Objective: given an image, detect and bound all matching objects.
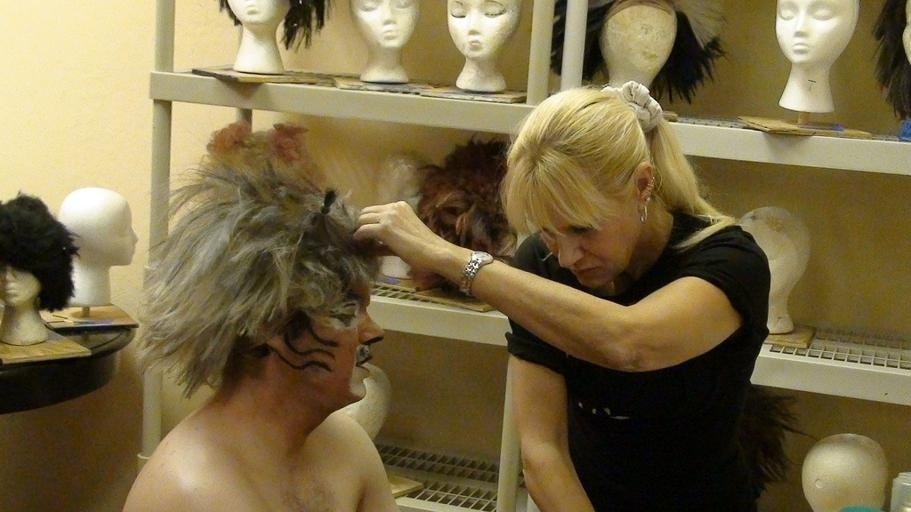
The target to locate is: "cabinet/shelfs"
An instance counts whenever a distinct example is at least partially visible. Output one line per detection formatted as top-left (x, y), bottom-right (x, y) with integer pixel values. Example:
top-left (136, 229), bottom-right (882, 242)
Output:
top-left (134, 1), bottom-right (555, 512)
top-left (536, 0), bottom-right (909, 512)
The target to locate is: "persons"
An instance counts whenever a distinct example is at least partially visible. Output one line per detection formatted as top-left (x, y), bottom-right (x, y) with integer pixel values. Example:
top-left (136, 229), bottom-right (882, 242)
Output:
top-left (123, 148), bottom-right (399, 512)
top-left (347, 2), bottom-right (419, 83)
top-left (551, 2), bottom-right (717, 107)
top-left (354, 82), bottom-right (770, 511)
top-left (219, 0), bottom-right (340, 76)
top-left (876, 0), bottom-right (910, 120)
top-left (775, 1), bottom-right (860, 113)
top-left (0, 195), bottom-right (75, 345)
top-left (445, 0), bottom-right (521, 94)
top-left (408, 141), bottom-right (517, 310)
top-left (741, 204), bottom-right (810, 336)
top-left (58, 185), bottom-right (135, 307)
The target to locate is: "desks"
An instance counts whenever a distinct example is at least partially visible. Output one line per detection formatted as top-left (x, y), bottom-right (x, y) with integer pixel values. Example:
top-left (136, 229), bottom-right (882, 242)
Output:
top-left (0, 304), bottom-right (136, 414)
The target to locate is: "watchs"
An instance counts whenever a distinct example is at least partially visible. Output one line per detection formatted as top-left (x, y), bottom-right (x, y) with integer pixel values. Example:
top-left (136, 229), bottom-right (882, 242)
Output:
top-left (460, 249), bottom-right (493, 296)
